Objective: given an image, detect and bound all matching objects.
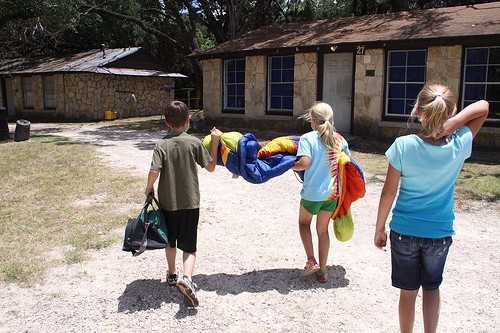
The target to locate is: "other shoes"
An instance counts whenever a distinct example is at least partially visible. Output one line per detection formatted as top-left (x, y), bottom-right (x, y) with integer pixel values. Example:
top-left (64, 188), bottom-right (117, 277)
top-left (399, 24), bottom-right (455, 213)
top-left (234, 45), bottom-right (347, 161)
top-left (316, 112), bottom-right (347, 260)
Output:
top-left (166, 266), bottom-right (180, 286)
top-left (176, 275), bottom-right (199, 307)
top-left (301, 257), bottom-right (320, 277)
top-left (316, 269), bottom-right (328, 283)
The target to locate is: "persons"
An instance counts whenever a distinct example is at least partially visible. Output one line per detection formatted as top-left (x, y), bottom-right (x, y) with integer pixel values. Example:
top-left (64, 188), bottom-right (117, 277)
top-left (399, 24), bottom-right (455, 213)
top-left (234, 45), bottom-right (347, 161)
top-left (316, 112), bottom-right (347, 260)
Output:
top-left (288, 103), bottom-right (351, 283)
top-left (145, 101), bottom-right (223, 308)
top-left (374, 83), bottom-right (489, 333)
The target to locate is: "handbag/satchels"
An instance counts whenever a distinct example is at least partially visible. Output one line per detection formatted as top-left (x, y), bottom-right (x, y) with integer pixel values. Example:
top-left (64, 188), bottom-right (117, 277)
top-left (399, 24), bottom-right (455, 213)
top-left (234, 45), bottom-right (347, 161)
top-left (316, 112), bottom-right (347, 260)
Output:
top-left (122, 192), bottom-right (168, 257)
top-left (333, 205), bottom-right (354, 242)
